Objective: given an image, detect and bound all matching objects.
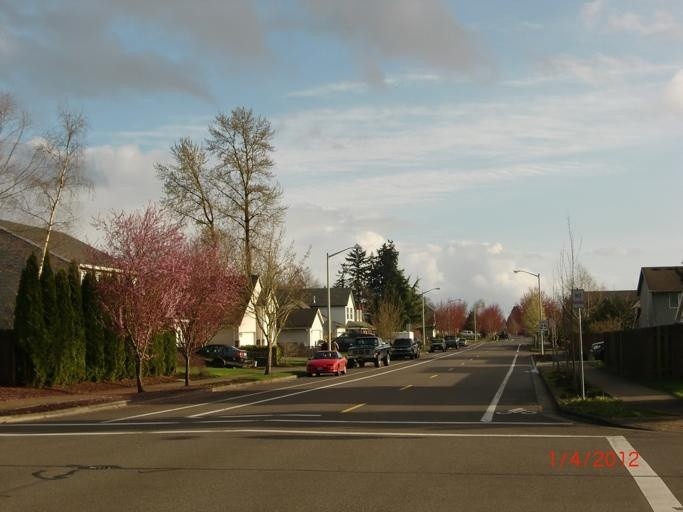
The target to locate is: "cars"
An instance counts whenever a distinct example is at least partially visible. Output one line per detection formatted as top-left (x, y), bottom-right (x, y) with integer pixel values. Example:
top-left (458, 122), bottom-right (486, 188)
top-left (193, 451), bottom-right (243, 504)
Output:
top-left (590, 341), bottom-right (604, 360)
top-left (194, 343), bottom-right (248, 369)
top-left (498, 331), bottom-right (509, 340)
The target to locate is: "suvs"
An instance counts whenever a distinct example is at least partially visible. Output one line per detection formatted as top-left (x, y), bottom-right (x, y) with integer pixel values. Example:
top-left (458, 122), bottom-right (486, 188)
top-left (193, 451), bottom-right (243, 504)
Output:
top-left (304, 330), bottom-right (481, 378)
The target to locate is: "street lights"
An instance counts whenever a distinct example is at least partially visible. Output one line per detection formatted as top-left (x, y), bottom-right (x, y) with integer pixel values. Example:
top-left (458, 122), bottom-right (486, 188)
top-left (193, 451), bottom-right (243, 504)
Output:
top-left (325, 245), bottom-right (361, 356)
top-left (513, 268), bottom-right (540, 356)
top-left (448, 299), bottom-right (461, 337)
top-left (421, 287), bottom-right (441, 345)
